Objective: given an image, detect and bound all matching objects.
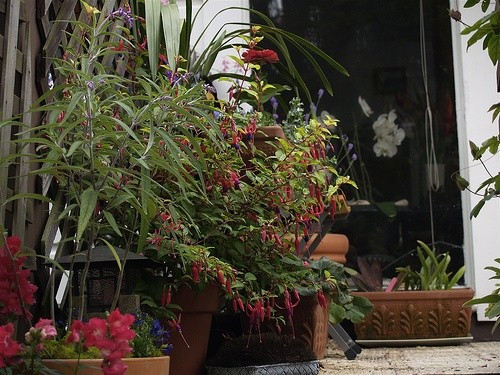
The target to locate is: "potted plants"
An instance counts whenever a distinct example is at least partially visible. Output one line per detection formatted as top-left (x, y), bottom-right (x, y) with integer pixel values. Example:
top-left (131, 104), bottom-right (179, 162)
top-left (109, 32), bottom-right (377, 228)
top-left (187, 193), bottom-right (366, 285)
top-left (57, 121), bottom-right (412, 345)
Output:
top-left (346, 239), bottom-right (474, 347)
